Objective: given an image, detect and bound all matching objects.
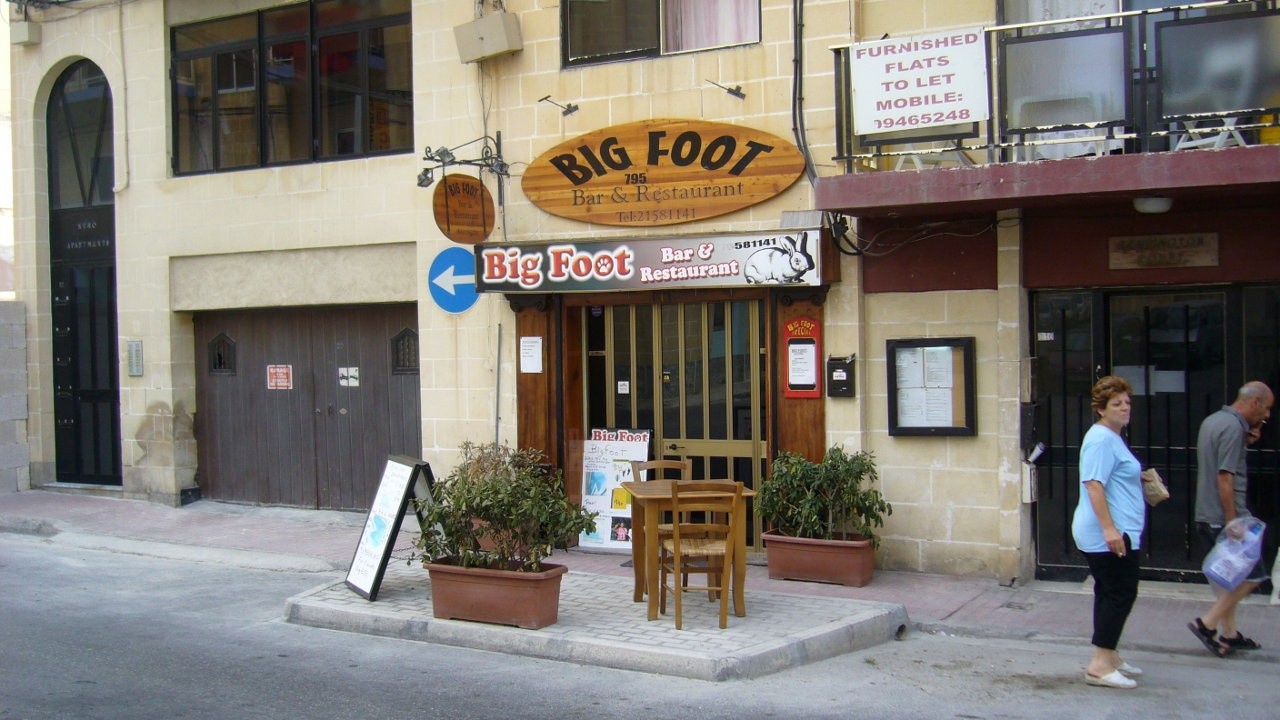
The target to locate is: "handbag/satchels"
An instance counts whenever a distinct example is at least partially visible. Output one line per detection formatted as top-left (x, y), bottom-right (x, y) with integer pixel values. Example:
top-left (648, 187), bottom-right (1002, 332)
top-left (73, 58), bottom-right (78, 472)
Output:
top-left (1202, 516), bottom-right (1267, 592)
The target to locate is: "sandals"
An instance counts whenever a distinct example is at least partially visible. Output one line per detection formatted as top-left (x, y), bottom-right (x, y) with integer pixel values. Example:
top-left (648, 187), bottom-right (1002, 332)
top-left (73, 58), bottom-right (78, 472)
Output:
top-left (1187, 616), bottom-right (1228, 659)
top-left (1218, 630), bottom-right (1262, 651)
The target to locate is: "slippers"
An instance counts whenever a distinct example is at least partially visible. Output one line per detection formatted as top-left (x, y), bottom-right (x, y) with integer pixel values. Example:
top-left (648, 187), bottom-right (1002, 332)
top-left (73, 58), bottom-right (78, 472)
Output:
top-left (1085, 669), bottom-right (1137, 689)
top-left (1115, 661), bottom-right (1143, 678)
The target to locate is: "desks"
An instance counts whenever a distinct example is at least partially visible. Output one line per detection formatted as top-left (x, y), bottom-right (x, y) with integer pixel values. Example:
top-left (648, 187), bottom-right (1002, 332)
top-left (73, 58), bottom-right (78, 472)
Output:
top-left (620, 479), bottom-right (759, 621)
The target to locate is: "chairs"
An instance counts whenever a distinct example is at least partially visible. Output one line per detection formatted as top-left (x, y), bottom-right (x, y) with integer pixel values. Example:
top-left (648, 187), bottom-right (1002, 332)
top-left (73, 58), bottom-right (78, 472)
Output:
top-left (627, 457), bottom-right (744, 629)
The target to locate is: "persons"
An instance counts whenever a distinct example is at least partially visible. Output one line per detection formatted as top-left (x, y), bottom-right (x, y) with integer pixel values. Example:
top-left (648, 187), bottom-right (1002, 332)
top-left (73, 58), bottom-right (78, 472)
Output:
top-left (1072, 375), bottom-right (1163, 689)
top-left (1186, 382), bottom-right (1275, 658)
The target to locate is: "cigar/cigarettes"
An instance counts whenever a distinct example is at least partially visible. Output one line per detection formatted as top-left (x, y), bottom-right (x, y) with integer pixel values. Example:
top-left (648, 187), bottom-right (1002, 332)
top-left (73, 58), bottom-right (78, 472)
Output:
top-left (1262, 420), bottom-right (1266, 423)
top-left (1119, 553), bottom-right (1122, 556)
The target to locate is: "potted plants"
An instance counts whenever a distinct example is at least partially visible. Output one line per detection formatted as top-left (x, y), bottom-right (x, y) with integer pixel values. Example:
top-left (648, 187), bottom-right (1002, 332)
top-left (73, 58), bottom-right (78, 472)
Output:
top-left (751, 442), bottom-right (893, 587)
top-left (407, 439), bottom-right (599, 628)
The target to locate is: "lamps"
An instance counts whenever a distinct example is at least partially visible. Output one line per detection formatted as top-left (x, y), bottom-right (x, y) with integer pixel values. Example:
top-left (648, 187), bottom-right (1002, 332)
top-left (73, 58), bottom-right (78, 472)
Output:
top-left (416, 130), bottom-right (509, 206)
top-left (1133, 198), bottom-right (1173, 212)
